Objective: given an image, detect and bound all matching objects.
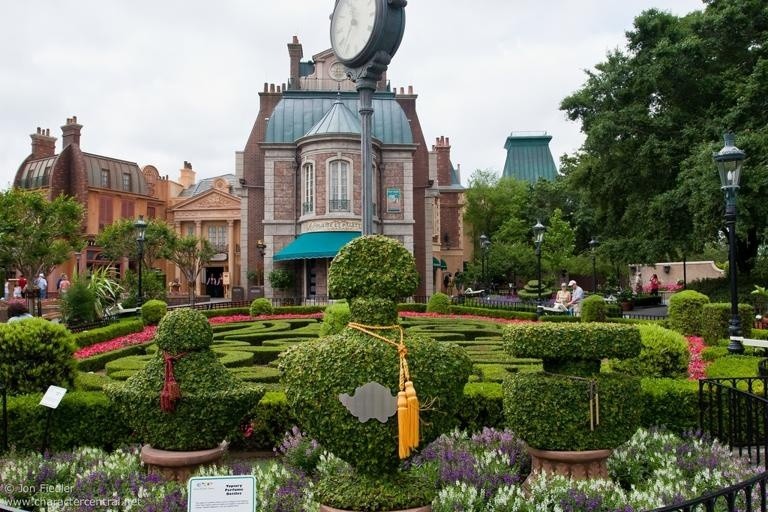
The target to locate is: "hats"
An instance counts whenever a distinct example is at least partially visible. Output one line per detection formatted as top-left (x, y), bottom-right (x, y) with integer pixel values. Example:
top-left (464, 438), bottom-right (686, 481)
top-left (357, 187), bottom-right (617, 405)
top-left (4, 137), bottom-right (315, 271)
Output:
top-left (561, 283), bottom-right (567, 286)
top-left (568, 280), bottom-right (576, 286)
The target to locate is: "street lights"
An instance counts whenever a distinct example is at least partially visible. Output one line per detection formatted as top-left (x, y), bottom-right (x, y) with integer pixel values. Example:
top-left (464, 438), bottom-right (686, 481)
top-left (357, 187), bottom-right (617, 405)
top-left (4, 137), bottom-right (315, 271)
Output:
top-left (713, 131), bottom-right (747, 356)
top-left (478, 233), bottom-right (487, 281)
top-left (255, 239), bottom-right (266, 288)
top-left (484, 240), bottom-right (490, 281)
top-left (531, 218), bottom-right (545, 318)
top-left (133, 215), bottom-right (147, 306)
top-left (74, 251), bottom-right (82, 279)
top-left (679, 246), bottom-right (686, 292)
top-left (585, 235), bottom-right (598, 294)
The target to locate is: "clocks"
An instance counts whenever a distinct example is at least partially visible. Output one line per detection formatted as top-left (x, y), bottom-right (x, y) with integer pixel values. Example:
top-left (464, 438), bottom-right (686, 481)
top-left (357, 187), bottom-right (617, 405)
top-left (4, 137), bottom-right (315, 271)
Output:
top-left (328, 0), bottom-right (406, 234)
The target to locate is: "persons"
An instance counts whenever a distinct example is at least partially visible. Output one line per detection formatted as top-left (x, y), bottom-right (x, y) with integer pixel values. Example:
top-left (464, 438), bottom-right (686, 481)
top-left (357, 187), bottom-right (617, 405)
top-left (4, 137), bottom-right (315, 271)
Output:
top-left (448, 272), bottom-right (454, 296)
top-left (565, 279), bottom-right (584, 317)
top-left (649, 274), bottom-right (659, 296)
top-left (34, 273), bottom-right (47, 298)
top-left (59, 275), bottom-right (71, 293)
top-left (18, 274), bottom-right (27, 298)
top-left (443, 275), bottom-right (449, 294)
top-left (454, 268), bottom-right (464, 295)
top-left (556, 283), bottom-right (572, 315)
top-left (56, 272), bottom-right (66, 295)
top-left (634, 272), bottom-right (644, 293)
